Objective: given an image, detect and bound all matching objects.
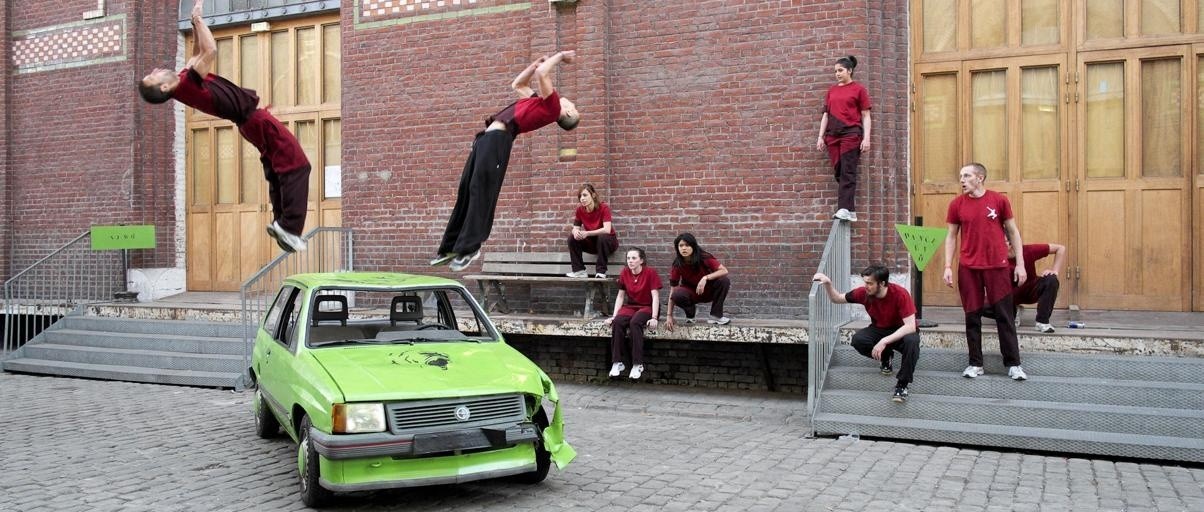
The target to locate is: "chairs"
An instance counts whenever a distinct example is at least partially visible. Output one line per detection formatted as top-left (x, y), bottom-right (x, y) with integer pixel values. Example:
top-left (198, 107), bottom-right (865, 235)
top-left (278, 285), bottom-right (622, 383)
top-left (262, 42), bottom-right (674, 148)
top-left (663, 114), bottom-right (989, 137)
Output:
top-left (380, 295), bottom-right (440, 331)
top-left (308, 295), bottom-right (369, 345)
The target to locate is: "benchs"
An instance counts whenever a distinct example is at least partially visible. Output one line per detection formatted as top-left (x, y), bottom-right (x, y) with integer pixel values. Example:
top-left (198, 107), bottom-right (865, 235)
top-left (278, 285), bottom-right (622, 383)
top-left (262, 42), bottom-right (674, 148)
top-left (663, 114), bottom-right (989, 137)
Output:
top-left (462, 251), bottom-right (627, 320)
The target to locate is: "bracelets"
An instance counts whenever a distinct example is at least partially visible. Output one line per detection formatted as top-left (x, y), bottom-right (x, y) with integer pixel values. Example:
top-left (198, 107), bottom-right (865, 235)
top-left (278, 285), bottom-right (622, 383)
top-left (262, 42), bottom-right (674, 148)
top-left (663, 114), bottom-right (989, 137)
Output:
top-left (944, 265), bottom-right (952, 269)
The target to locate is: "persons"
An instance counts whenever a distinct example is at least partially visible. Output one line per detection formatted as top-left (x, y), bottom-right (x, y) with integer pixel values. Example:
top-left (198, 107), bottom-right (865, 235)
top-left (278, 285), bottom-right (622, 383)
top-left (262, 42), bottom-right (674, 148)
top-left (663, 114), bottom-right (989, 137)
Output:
top-left (568, 183), bottom-right (619, 279)
top-left (601, 249), bottom-right (662, 381)
top-left (811, 264), bottom-right (919, 406)
top-left (140, 1), bottom-right (311, 254)
top-left (975, 228), bottom-right (1065, 333)
top-left (943, 162), bottom-right (1029, 383)
top-left (431, 48), bottom-right (580, 272)
top-left (817, 55), bottom-right (871, 222)
top-left (666, 232), bottom-right (732, 331)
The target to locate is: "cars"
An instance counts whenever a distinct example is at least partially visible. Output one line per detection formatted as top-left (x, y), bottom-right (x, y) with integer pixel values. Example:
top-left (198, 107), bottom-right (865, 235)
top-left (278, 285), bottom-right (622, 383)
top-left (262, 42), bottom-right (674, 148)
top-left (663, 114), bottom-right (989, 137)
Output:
top-left (246, 271), bottom-right (577, 506)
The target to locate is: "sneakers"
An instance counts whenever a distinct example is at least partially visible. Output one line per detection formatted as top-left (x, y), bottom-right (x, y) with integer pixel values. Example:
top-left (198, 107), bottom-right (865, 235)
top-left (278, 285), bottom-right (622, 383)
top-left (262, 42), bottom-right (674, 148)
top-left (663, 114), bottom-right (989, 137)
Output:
top-left (628, 365), bottom-right (644, 379)
top-left (449, 248), bottom-right (481, 272)
top-left (962, 365), bottom-right (985, 378)
top-left (707, 312), bottom-right (731, 325)
top-left (267, 221), bottom-right (305, 253)
top-left (1035, 321), bottom-right (1054, 333)
top-left (1007, 366), bottom-right (1027, 380)
top-left (832, 208), bottom-right (857, 222)
top-left (566, 270), bottom-right (588, 278)
top-left (595, 273), bottom-right (606, 278)
top-left (609, 362), bottom-right (625, 377)
top-left (881, 351), bottom-right (894, 374)
top-left (893, 386), bottom-right (908, 402)
top-left (430, 252), bottom-right (459, 266)
top-left (686, 317), bottom-right (695, 323)
top-left (1014, 305), bottom-right (1024, 327)
